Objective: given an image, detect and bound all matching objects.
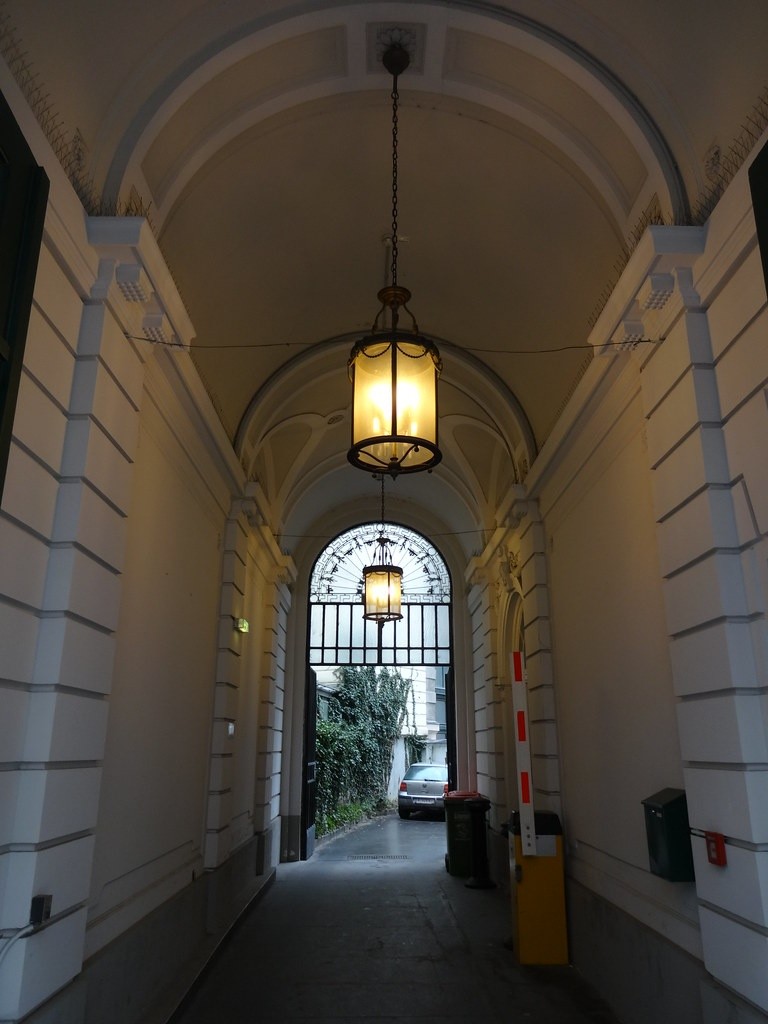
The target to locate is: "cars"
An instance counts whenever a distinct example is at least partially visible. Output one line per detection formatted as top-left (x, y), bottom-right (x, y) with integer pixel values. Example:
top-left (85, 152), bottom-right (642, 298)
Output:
top-left (398, 760), bottom-right (450, 818)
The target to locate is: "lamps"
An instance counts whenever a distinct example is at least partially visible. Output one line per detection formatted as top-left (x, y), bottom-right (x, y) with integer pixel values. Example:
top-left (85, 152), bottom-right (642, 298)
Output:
top-left (234, 618), bottom-right (249, 634)
top-left (363, 473), bottom-right (404, 627)
top-left (346, 28), bottom-right (443, 481)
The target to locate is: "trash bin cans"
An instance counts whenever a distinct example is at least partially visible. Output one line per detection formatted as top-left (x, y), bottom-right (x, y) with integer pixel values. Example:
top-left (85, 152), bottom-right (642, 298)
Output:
top-left (441, 791), bottom-right (492, 878)
